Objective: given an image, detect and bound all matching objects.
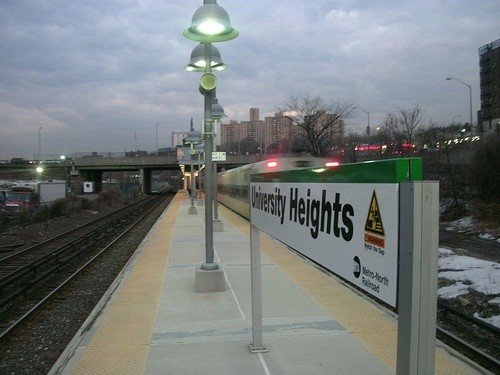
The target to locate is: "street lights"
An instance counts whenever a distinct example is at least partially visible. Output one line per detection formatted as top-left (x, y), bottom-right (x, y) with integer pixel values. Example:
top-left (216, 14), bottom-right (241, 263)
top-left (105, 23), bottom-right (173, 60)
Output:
top-left (445, 77), bottom-right (473, 142)
top-left (450, 115), bottom-right (463, 145)
top-left (352, 106), bottom-right (371, 161)
top-left (183, 0), bottom-right (240, 293)
top-left (38, 126), bottom-right (42, 161)
top-left (155, 122), bottom-right (160, 154)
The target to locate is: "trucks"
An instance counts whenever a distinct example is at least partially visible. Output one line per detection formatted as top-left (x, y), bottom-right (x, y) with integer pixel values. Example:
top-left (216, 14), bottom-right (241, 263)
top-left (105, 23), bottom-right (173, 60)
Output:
top-left (0, 178), bottom-right (66, 224)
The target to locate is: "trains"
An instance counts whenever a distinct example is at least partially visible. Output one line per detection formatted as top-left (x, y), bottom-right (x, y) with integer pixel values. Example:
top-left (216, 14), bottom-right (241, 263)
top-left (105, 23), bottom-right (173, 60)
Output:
top-left (195, 156), bottom-right (341, 221)
